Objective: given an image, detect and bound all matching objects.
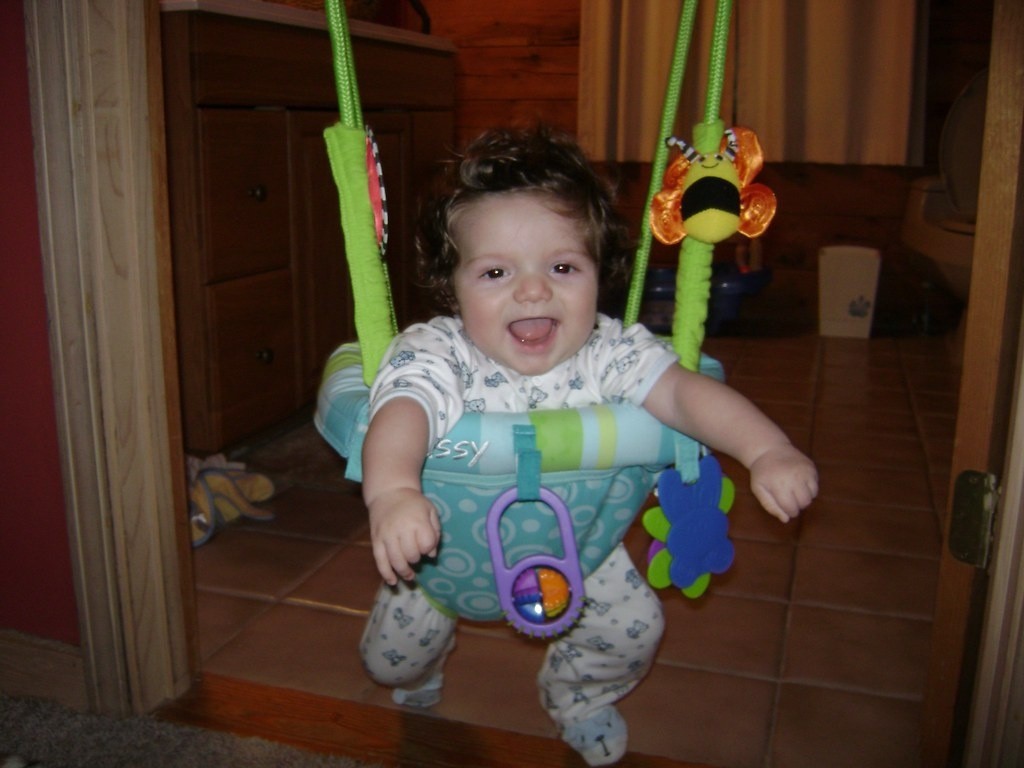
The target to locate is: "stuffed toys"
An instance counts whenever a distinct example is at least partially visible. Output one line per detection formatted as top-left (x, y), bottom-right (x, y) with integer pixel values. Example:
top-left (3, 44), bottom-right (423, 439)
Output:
top-left (651, 128), bottom-right (777, 244)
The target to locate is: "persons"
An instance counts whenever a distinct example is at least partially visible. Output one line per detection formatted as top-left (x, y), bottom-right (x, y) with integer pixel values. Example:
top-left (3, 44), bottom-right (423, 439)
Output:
top-left (360, 126), bottom-right (818, 768)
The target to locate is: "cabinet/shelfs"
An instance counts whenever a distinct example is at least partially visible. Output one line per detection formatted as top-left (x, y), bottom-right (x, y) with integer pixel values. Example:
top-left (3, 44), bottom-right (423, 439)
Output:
top-left (401, 110), bottom-right (459, 325)
top-left (283, 111), bottom-right (412, 411)
top-left (194, 104), bottom-right (307, 455)
